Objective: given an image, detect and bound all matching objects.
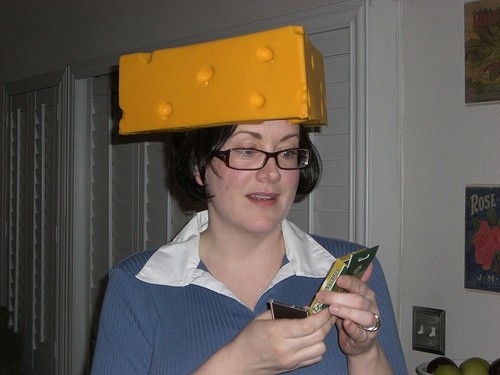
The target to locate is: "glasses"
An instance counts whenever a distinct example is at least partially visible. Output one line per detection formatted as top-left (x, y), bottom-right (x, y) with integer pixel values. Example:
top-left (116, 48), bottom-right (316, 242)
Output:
top-left (215, 146), bottom-right (311, 170)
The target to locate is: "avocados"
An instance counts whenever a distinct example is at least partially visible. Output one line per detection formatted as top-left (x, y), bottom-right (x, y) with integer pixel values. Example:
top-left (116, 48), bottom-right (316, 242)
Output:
top-left (425, 356), bottom-right (500, 375)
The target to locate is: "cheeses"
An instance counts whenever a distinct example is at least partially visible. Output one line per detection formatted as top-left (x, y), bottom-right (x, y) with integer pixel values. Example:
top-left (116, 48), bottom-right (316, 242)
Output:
top-left (117, 25), bottom-right (327, 134)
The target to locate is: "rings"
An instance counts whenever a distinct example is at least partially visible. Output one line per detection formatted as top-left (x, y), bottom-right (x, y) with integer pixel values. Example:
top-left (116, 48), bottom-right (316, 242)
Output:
top-left (363, 314), bottom-right (380, 331)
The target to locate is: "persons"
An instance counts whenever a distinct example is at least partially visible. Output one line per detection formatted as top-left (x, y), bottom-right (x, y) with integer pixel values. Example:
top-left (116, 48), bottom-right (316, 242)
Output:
top-left (90, 118), bottom-right (408, 375)
